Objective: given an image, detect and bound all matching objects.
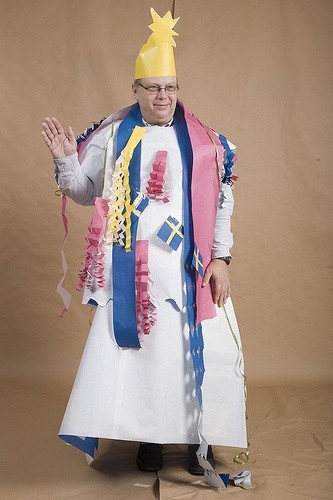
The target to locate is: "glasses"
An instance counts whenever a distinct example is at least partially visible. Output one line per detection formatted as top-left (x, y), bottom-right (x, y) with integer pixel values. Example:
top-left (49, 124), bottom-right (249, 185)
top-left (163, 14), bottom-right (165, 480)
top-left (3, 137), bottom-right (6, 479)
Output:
top-left (136, 82), bottom-right (180, 94)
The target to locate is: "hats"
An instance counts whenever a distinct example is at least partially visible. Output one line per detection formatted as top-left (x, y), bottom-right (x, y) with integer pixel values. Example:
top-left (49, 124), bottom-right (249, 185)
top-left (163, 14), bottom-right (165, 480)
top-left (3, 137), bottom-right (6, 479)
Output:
top-left (134, 8), bottom-right (180, 81)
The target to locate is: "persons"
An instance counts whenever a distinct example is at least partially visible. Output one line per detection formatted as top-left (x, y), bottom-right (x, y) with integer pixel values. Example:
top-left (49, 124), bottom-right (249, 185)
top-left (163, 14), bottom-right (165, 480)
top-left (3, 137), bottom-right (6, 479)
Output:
top-left (41, 6), bottom-right (247, 476)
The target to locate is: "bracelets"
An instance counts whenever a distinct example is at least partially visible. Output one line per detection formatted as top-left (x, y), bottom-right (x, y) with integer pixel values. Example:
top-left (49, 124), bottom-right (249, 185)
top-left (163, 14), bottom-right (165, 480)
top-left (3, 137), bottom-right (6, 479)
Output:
top-left (211, 255), bottom-right (231, 265)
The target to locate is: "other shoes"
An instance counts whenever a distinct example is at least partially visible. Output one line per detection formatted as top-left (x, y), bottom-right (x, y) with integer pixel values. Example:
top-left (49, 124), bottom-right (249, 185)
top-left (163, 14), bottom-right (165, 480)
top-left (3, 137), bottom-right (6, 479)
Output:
top-left (188, 444), bottom-right (216, 475)
top-left (137, 442), bottom-right (165, 472)
